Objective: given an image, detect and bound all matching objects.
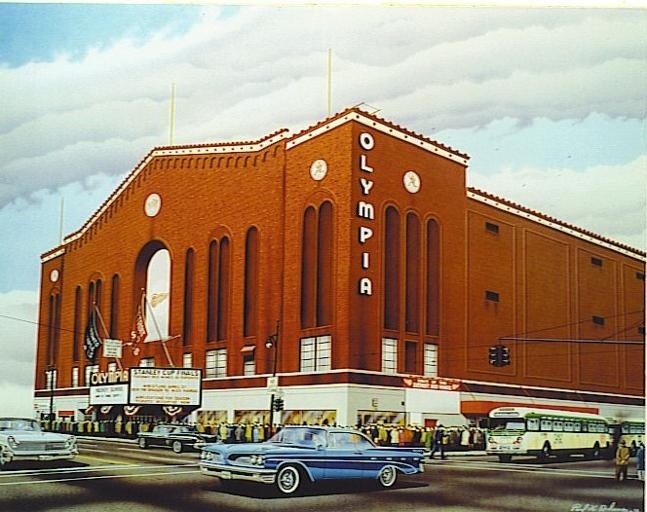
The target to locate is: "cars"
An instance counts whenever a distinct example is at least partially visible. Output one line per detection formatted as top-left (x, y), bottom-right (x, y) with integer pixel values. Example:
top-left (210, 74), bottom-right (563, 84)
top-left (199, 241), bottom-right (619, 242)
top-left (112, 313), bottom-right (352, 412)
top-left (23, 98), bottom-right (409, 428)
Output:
top-left (137, 422), bottom-right (221, 455)
top-left (198, 424), bottom-right (430, 489)
top-left (0, 415), bottom-right (80, 470)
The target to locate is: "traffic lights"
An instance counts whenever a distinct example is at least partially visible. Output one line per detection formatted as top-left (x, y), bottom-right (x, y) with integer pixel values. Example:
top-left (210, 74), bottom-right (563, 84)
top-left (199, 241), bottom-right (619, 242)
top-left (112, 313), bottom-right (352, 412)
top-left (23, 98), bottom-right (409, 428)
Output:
top-left (499, 346), bottom-right (510, 366)
top-left (488, 345), bottom-right (498, 366)
top-left (273, 398), bottom-right (285, 413)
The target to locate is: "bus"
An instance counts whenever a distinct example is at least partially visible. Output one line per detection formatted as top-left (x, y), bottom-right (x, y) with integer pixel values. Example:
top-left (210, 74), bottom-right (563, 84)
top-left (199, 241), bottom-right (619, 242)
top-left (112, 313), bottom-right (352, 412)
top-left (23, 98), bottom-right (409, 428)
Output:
top-left (485, 407), bottom-right (610, 460)
top-left (602, 415), bottom-right (645, 456)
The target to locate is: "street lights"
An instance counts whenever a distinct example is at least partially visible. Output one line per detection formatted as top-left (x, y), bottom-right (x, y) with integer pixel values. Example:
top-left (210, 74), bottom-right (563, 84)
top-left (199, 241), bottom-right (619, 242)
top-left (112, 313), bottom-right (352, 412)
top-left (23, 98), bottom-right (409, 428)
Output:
top-left (265, 319), bottom-right (281, 440)
top-left (45, 365), bottom-right (58, 430)
